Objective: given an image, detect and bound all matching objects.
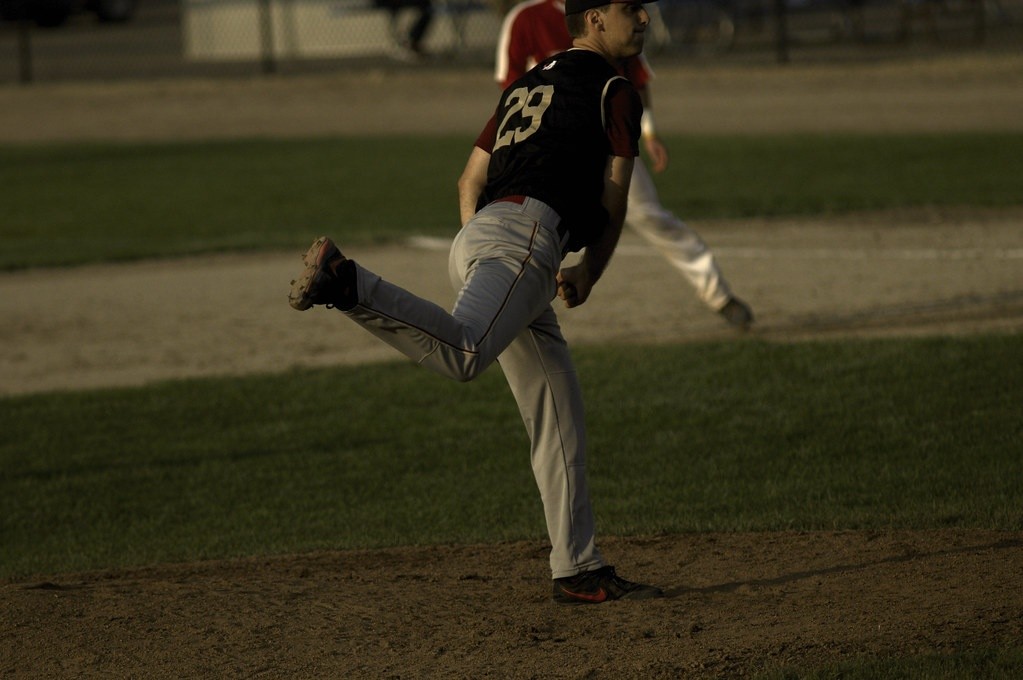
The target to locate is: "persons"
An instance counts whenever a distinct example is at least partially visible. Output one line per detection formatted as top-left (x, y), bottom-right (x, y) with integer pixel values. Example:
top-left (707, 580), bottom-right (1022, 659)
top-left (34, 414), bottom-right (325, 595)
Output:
top-left (495, 0), bottom-right (753, 329)
top-left (288, 0), bottom-right (665, 607)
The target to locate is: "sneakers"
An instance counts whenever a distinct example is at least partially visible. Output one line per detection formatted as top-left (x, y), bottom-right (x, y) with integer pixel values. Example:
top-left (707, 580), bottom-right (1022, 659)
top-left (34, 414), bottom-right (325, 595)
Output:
top-left (552, 564), bottom-right (663, 604)
top-left (287, 236), bottom-right (347, 311)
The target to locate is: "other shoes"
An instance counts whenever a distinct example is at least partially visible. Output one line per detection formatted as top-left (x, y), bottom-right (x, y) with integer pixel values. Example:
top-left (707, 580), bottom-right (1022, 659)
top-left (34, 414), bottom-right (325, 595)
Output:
top-left (721, 298), bottom-right (755, 331)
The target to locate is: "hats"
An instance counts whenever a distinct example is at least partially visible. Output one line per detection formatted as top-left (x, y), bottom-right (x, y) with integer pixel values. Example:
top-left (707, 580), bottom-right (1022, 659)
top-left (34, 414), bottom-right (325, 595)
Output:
top-left (565, 0), bottom-right (659, 16)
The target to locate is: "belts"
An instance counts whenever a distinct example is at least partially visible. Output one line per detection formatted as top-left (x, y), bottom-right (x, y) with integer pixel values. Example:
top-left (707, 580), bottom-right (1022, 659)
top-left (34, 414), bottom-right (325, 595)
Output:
top-left (494, 194), bottom-right (567, 241)
